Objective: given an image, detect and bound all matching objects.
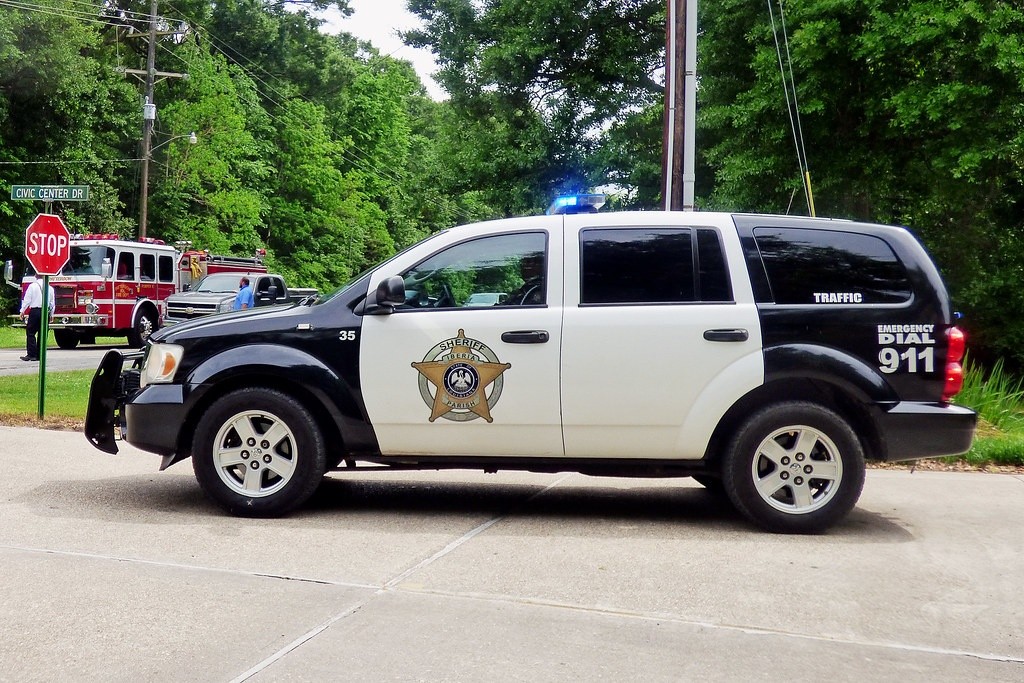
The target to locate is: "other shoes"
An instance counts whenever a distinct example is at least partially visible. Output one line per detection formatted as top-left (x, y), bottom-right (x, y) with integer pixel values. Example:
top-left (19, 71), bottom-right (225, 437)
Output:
top-left (21, 355), bottom-right (36, 361)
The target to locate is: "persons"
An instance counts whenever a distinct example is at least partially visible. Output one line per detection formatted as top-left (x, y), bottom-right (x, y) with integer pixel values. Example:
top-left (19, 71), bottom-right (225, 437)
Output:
top-left (494, 252), bottom-right (544, 306)
top-left (20, 273), bottom-right (56, 361)
top-left (234, 277), bottom-right (254, 310)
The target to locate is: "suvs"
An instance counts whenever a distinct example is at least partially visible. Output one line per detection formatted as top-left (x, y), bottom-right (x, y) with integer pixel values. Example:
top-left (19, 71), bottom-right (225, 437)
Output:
top-left (85, 193), bottom-right (982, 536)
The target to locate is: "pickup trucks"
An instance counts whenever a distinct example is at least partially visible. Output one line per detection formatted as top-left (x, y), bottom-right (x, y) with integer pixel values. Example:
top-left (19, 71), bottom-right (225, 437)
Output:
top-left (158, 272), bottom-right (320, 331)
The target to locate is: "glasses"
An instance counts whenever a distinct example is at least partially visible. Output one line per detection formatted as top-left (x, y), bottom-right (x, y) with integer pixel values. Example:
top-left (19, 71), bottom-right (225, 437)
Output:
top-left (519, 260), bottom-right (537, 268)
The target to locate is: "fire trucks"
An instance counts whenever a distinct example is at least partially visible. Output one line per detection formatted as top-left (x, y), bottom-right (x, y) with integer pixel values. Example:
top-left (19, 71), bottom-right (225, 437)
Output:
top-left (4, 233), bottom-right (268, 350)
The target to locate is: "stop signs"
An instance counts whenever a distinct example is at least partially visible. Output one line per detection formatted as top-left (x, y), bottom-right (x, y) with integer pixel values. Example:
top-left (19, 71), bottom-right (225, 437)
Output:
top-left (25, 213), bottom-right (71, 275)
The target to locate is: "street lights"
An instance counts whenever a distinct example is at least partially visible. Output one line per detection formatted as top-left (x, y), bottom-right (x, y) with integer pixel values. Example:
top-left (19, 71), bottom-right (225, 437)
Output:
top-left (138, 131), bottom-right (198, 237)
